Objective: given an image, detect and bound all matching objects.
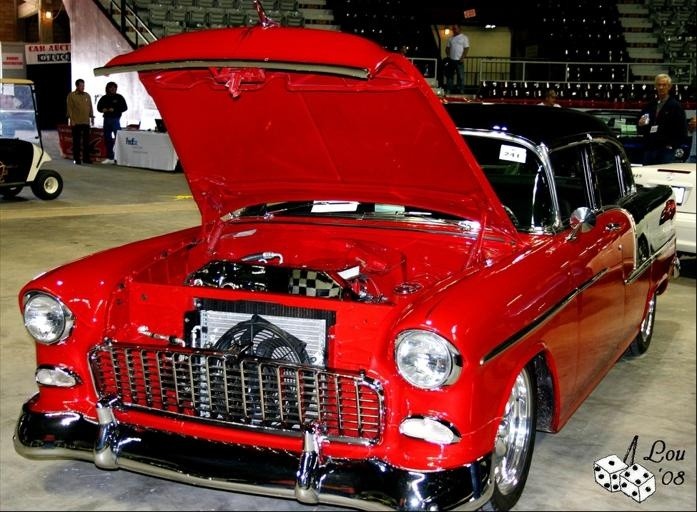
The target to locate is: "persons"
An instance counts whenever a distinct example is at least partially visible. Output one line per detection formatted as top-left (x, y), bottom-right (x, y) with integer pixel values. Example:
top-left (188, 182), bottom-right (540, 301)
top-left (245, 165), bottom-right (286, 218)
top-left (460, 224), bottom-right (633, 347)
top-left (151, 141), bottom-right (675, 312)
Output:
top-left (686, 118), bottom-right (696, 161)
top-left (535, 88), bottom-right (564, 108)
top-left (97, 82), bottom-right (128, 164)
top-left (66, 79), bottom-right (94, 164)
top-left (636, 73), bottom-right (686, 164)
top-left (443, 24), bottom-right (470, 94)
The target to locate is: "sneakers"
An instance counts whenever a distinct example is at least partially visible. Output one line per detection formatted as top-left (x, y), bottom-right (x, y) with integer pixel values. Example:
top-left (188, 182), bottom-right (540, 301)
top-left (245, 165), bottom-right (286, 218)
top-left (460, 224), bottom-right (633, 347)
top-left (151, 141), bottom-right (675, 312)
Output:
top-left (72, 159), bottom-right (117, 165)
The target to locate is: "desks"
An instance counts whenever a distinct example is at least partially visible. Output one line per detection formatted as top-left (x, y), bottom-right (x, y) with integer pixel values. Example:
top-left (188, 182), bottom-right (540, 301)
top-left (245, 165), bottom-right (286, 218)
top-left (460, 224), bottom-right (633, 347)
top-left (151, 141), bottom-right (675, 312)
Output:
top-left (111, 127), bottom-right (182, 171)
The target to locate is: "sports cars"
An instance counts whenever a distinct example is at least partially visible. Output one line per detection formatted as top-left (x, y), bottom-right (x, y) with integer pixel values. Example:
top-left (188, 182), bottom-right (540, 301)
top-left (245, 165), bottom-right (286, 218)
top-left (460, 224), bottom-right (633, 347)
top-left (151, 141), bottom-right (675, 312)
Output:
top-left (14, 24), bottom-right (679, 512)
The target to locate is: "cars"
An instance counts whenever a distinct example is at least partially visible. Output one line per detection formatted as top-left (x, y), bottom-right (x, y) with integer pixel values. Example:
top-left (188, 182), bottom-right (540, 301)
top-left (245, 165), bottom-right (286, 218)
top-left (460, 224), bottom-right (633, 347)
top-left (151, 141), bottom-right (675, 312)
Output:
top-left (629, 164), bottom-right (696, 258)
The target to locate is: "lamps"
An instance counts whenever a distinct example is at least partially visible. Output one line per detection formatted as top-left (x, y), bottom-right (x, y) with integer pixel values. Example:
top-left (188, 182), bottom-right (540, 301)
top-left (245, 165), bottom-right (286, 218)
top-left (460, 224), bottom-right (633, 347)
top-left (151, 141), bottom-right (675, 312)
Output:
top-left (44, 7), bottom-right (53, 19)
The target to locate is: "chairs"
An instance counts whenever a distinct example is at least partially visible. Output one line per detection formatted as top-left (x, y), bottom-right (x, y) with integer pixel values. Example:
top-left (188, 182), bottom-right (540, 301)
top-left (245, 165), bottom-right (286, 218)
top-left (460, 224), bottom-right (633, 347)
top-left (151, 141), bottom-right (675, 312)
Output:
top-left (100, 1), bottom-right (341, 37)
top-left (617, 0), bottom-right (696, 80)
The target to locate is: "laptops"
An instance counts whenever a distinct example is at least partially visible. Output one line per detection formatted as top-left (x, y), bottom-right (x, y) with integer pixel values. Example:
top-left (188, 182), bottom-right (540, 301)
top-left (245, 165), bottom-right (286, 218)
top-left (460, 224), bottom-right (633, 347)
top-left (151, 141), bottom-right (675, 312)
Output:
top-left (154, 118), bottom-right (167, 132)
